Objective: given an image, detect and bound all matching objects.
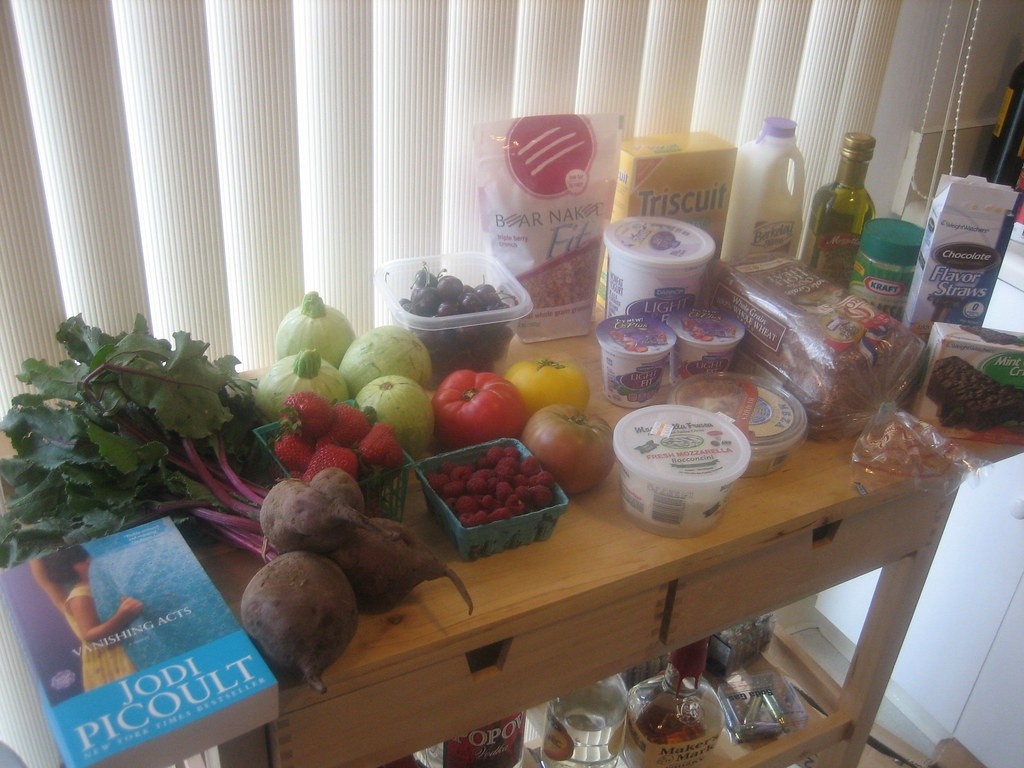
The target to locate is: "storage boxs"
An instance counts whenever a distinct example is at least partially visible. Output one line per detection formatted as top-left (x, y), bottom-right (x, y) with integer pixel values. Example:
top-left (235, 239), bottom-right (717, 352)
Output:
top-left (912, 322), bottom-right (1024, 444)
top-left (594, 131), bottom-right (737, 308)
top-left (252, 400), bottom-right (414, 520)
top-left (378, 253), bottom-right (534, 376)
top-left (903, 173), bottom-right (1024, 342)
top-left (413, 437), bottom-right (568, 562)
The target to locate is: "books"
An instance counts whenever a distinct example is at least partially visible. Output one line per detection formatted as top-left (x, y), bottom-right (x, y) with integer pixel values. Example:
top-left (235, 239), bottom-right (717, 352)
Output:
top-left (0, 515), bottom-right (279, 768)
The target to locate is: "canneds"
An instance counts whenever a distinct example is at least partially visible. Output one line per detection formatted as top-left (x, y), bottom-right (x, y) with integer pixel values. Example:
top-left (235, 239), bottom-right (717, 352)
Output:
top-left (849, 217), bottom-right (926, 323)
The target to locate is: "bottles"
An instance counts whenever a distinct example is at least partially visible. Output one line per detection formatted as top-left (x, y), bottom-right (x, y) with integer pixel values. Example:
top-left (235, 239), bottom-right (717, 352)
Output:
top-left (719, 116), bottom-right (805, 264)
top-left (415, 708), bottom-right (526, 768)
top-left (980, 60), bottom-right (1024, 186)
top-left (540, 673), bottom-right (628, 768)
top-left (799, 131), bottom-right (876, 294)
top-left (620, 636), bottom-right (725, 768)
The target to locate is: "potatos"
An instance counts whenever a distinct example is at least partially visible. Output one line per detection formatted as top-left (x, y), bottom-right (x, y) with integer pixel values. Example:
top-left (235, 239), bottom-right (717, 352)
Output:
top-left (238, 467), bottom-right (477, 693)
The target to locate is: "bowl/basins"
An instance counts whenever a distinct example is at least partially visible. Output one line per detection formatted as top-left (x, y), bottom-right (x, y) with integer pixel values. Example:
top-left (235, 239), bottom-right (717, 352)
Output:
top-left (381, 252), bottom-right (534, 385)
top-left (613, 404), bottom-right (752, 539)
top-left (669, 372), bottom-right (808, 478)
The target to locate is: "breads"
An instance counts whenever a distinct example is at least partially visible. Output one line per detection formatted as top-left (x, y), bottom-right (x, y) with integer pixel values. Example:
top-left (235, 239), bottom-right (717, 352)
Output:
top-left (697, 252), bottom-right (923, 444)
top-left (928, 356), bottom-right (1023, 432)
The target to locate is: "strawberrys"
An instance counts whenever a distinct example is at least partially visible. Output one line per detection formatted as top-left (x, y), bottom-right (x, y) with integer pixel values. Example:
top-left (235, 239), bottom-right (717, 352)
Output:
top-left (266, 389), bottom-right (402, 489)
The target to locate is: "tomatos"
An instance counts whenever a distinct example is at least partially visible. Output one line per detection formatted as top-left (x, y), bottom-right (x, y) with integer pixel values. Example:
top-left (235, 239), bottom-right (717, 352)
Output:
top-left (429, 357), bottom-right (616, 495)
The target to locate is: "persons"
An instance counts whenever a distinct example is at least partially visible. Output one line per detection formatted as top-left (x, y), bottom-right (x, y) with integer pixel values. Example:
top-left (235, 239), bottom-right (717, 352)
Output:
top-left (42, 543), bottom-right (142, 693)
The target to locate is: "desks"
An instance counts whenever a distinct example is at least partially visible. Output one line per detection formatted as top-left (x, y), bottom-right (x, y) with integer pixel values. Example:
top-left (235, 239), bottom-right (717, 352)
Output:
top-left (179, 289), bottom-right (1024, 768)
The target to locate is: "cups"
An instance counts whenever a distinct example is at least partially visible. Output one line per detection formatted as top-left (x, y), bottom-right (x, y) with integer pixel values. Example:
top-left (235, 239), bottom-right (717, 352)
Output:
top-left (664, 307), bottom-right (744, 388)
top-left (604, 216), bottom-right (716, 326)
top-left (596, 315), bottom-right (677, 408)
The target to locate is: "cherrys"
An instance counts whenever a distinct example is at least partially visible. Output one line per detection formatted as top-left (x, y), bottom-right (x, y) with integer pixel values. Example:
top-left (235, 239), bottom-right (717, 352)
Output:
top-left (397, 261), bottom-right (519, 382)
